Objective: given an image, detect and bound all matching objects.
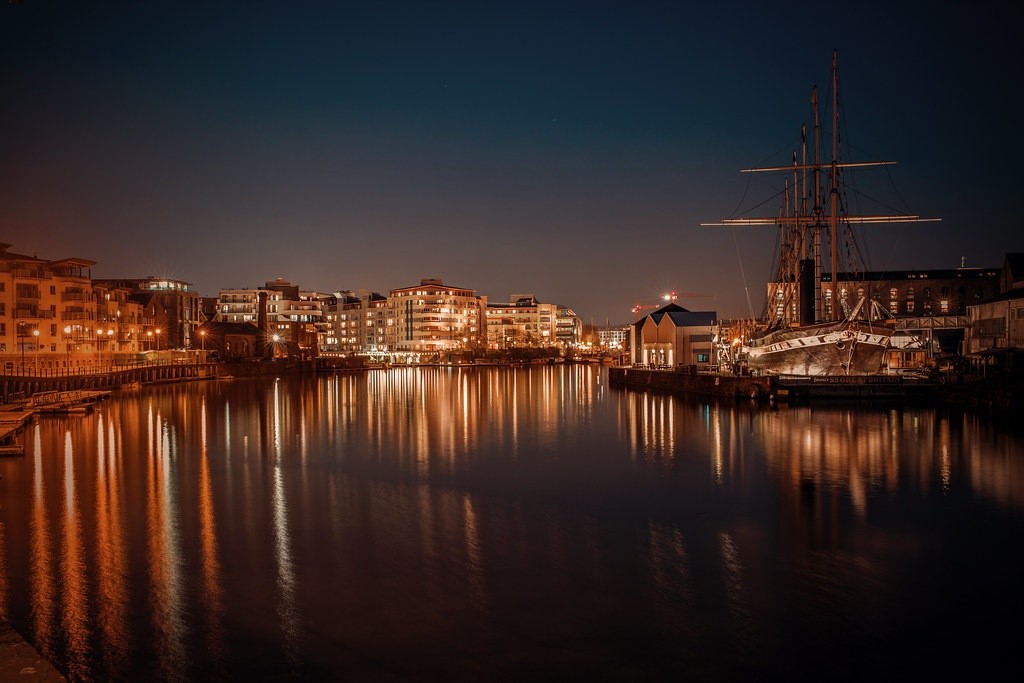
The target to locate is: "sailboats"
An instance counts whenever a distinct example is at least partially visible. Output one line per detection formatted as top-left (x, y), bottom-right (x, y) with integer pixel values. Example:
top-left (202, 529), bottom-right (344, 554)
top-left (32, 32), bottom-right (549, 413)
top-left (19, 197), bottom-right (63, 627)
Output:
top-left (700, 44), bottom-right (943, 399)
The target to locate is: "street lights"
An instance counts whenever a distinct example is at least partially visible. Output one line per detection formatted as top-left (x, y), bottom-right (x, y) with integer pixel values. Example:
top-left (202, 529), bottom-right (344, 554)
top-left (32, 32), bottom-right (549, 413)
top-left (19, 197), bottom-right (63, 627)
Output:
top-left (33, 330), bottom-right (40, 373)
top-left (200, 329), bottom-right (207, 350)
top-left (147, 331), bottom-right (152, 351)
top-left (107, 329), bottom-right (115, 366)
top-left (18, 321), bottom-right (25, 377)
top-left (64, 326), bottom-right (73, 371)
top-left (156, 328), bottom-right (161, 365)
top-left (97, 329), bottom-right (104, 366)
